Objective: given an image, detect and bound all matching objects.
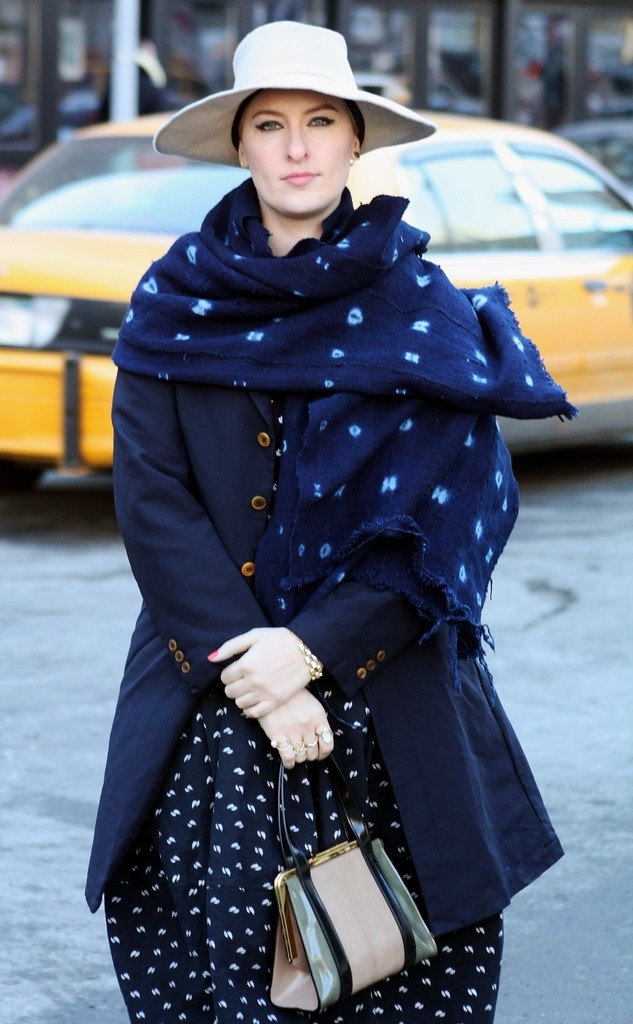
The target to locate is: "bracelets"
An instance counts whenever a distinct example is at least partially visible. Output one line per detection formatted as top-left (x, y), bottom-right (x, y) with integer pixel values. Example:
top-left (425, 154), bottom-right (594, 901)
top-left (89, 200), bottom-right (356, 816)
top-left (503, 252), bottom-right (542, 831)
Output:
top-left (291, 631), bottom-right (323, 680)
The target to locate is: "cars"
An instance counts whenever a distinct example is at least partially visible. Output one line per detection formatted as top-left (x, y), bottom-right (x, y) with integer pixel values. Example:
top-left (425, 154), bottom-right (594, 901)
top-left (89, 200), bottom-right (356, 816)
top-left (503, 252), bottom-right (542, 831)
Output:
top-left (1, 106), bottom-right (633, 499)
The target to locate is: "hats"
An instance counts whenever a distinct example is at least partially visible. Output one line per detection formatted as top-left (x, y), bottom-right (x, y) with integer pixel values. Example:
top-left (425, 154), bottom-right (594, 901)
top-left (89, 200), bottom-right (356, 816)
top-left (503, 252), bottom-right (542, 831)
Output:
top-left (153, 21), bottom-right (436, 167)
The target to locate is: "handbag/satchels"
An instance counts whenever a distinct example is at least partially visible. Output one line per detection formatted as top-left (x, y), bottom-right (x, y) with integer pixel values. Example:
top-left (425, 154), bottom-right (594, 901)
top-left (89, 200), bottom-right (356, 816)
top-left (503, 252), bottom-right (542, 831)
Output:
top-left (271, 753), bottom-right (436, 1012)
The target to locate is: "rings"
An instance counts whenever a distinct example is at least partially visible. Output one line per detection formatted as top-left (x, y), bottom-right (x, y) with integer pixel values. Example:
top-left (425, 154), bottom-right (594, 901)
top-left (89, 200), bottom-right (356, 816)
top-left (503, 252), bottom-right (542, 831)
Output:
top-left (271, 728), bottom-right (334, 756)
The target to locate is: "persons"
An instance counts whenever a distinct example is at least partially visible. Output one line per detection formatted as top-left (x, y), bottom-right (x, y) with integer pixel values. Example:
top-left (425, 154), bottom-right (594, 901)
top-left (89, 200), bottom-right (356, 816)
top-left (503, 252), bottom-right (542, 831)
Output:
top-left (86, 20), bottom-right (578, 1024)
